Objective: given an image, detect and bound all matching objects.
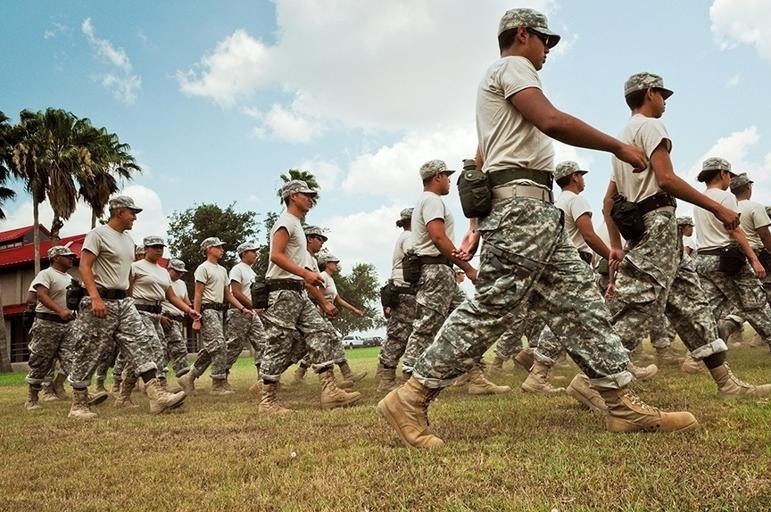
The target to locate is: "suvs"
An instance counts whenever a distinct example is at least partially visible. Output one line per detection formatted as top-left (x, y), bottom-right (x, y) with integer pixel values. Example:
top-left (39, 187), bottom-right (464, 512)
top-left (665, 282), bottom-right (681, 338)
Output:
top-left (341, 334), bottom-right (363, 350)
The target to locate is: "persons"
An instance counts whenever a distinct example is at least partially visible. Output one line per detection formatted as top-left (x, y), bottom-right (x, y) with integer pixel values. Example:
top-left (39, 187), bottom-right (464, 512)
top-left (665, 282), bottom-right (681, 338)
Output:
top-left (520, 160), bottom-right (658, 395)
top-left (110, 236), bottom-right (202, 409)
top-left (70, 196), bottom-right (185, 418)
top-left (250, 225), bottom-right (353, 402)
top-left (36, 360), bottom-right (71, 404)
top-left (225, 242), bottom-right (268, 392)
top-left (398, 160), bottom-right (511, 398)
top-left (728, 173), bottom-right (771, 302)
top-left (375, 208), bottom-right (415, 392)
top-left (374, 8), bottom-right (699, 451)
top-left (177, 237), bottom-right (253, 397)
top-left (25, 246), bottom-right (109, 411)
top-left (677, 215), bottom-right (697, 258)
top-left (565, 73), bottom-right (771, 412)
top-left (292, 254), bottom-right (368, 387)
top-left (26, 273), bottom-right (37, 305)
top-left (159, 257), bottom-right (194, 394)
top-left (682, 157), bottom-right (771, 377)
top-left (256, 180), bottom-right (361, 417)
top-left (451, 266), bottom-right (466, 285)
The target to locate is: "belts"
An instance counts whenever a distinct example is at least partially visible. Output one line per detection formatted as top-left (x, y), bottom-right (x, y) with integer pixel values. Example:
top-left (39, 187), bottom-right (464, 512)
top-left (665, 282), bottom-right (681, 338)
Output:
top-left (398, 286), bottom-right (416, 294)
top-left (491, 183), bottom-right (550, 203)
top-left (698, 247), bottom-right (721, 256)
top-left (638, 193), bottom-right (677, 214)
top-left (163, 312), bottom-right (183, 322)
top-left (134, 298), bottom-right (162, 306)
top-left (82, 287), bottom-right (127, 299)
top-left (419, 253), bottom-right (454, 267)
top-left (35, 311), bottom-right (76, 323)
top-left (201, 302), bottom-right (224, 311)
top-left (226, 303), bottom-right (236, 308)
top-left (488, 167), bottom-right (554, 189)
top-left (266, 279), bottom-right (304, 291)
top-left (134, 303), bottom-right (162, 314)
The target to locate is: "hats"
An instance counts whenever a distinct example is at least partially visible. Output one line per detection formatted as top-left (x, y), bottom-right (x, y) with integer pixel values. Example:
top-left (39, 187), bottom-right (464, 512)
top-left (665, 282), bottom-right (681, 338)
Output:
top-left (47, 245), bottom-right (77, 260)
top-left (554, 160), bottom-right (589, 181)
top-left (702, 157), bottom-right (737, 178)
top-left (168, 258), bottom-right (188, 272)
top-left (283, 180), bottom-right (317, 200)
top-left (135, 245), bottom-right (146, 255)
top-left (400, 208), bottom-right (414, 219)
top-left (237, 242), bottom-right (260, 255)
top-left (304, 226), bottom-right (328, 241)
top-left (419, 159), bottom-right (456, 181)
top-left (143, 235), bottom-right (167, 247)
top-left (109, 195), bottom-right (143, 214)
top-left (676, 216), bottom-right (695, 227)
top-left (199, 237), bottom-right (227, 251)
top-left (320, 254), bottom-right (340, 262)
top-left (729, 172), bottom-right (754, 189)
top-left (624, 72), bottom-right (673, 100)
top-left (498, 7), bottom-right (561, 49)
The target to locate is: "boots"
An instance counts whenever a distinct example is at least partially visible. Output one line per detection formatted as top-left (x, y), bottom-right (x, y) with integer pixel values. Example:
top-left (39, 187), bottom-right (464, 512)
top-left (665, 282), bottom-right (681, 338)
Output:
top-left (87, 392), bottom-right (108, 406)
top-left (319, 368), bottom-right (361, 410)
top-left (377, 374), bottom-right (444, 451)
top-left (289, 366), bottom-right (311, 386)
top-left (222, 370), bottom-right (236, 391)
top-left (95, 384), bottom-right (115, 401)
top-left (516, 347), bottom-right (537, 373)
top-left (375, 364), bottom-right (384, 382)
top-left (598, 384), bottom-right (698, 434)
top-left (565, 372), bottom-right (608, 413)
top-left (68, 387), bottom-right (98, 421)
top-left (626, 360), bottom-right (658, 380)
top-left (258, 383), bottom-right (291, 416)
top-left (680, 350), bottom-right (711, 376)
top-left (521, 360), bottom-right (566, 394)
top-left (176, 374), bottom-right (199, 397)
top-left (340, 362), bottom-right (367, 382)
top-left (468, 363), bottom-right (511, 394)
top-left (24, 383), bottom-right (44, 410)
top-left (400, 373), bottom-right (410, 385)
top-left (630, 342), bottom-right (654, 363)
top-left (159, 378), bottom-right (180, 393)
top-left (209, 378), bottom-right (231, 395)
top-left (112, 393), bottom-right (139, 409)
top-left (512, 358), bottom-right (527, 375)
top-left (144, 378), bottom-right (186, 415)
top-left (730, 328), bottom-right (744, 347)
top-left (750, 330), bottom-right (766, 348)
top-left (42, 385), bottom-right (59, 401)
top-left (110, 388), bottom-right (121, 398)
top-left (334, 377), bottom-right (354, 389)
top-left (709, 361), bottom-right (771, 399)
top-left (53, 372), bottom-right (69, 399)
top-left (487, 356), bottom-right (513, 377)
top-left (249, 380), bottom-right (263, 402)
top-left (377, 368), bottom-right (401, 393)
top-left (655, 346), bottom-right (686, 368)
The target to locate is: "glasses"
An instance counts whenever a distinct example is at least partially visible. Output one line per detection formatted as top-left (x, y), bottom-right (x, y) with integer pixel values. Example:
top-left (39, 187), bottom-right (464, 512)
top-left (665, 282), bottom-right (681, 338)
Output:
top-left (527, 28), bottom-right (549, 45)
top-left (312, 235), bottom-right (322, 241)
top-left (172, 268), bottom-right (182, 274)
